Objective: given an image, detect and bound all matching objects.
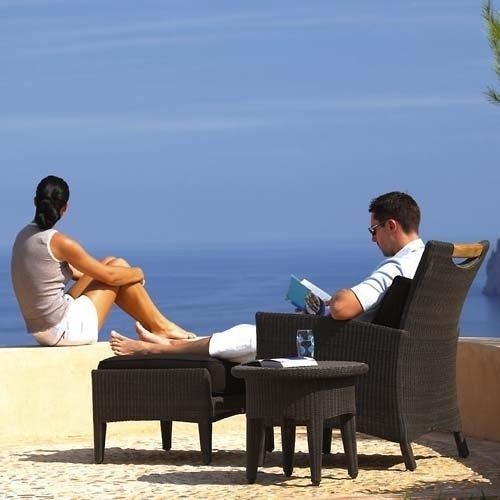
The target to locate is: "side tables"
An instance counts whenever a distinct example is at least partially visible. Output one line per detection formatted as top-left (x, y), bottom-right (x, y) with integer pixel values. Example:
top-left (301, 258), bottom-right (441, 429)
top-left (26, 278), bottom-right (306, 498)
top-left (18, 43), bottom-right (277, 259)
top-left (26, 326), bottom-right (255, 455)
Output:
top-left (232, 361), bottom-right (371, 487)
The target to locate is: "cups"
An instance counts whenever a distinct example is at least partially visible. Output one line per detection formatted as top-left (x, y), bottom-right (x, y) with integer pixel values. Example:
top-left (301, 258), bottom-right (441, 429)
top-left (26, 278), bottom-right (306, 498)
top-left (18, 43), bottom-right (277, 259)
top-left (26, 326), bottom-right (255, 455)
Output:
top-left (297, 330), bottom-right (315, 357)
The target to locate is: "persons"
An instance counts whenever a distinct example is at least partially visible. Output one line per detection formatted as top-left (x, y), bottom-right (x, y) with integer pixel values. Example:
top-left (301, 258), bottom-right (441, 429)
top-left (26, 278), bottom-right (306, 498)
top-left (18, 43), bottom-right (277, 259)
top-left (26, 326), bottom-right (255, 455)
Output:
top-left (109, 192), bottom-right (428, 365)
top-left (10, 175), bottom-right (197, 346)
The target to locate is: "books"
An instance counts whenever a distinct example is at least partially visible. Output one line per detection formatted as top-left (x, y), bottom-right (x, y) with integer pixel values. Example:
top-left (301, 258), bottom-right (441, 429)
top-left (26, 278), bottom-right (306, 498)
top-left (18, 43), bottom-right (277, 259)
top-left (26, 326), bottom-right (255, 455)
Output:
top-left (242, 356), bottom-right (318, 368)
top-left (285, 275), bottom-right (333, 317)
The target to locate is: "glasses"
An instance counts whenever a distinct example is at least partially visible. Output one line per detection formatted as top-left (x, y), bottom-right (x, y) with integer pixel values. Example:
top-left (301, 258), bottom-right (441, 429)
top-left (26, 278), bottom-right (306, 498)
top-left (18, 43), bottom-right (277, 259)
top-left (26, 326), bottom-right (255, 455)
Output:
top-left (368, 221), bottom-right (385, 234)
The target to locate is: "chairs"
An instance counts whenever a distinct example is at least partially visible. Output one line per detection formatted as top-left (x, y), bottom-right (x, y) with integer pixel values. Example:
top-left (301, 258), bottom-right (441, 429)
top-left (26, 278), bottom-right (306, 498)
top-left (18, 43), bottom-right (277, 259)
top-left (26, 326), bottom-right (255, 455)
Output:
top-left (259, 238), bottom-right (490, 473)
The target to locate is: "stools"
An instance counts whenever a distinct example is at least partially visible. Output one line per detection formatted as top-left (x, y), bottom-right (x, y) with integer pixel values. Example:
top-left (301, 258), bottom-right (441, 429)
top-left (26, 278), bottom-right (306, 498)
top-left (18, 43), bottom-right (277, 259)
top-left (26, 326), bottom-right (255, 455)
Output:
top-left (91, 351), bottom-right (244, 467)
